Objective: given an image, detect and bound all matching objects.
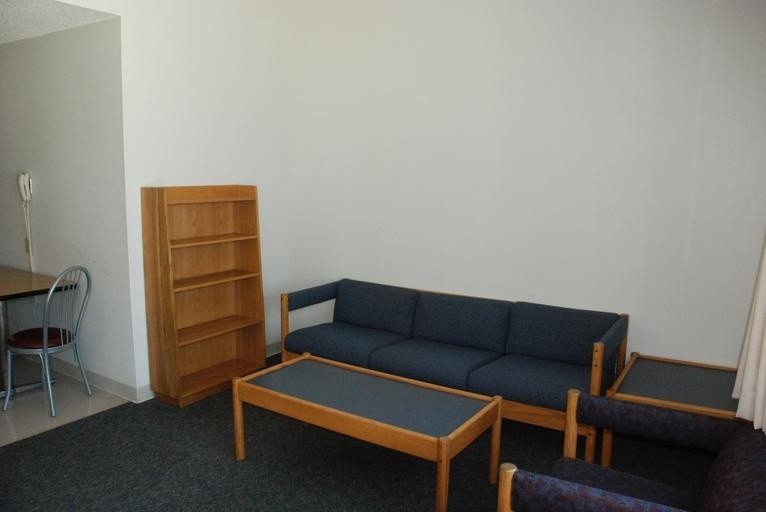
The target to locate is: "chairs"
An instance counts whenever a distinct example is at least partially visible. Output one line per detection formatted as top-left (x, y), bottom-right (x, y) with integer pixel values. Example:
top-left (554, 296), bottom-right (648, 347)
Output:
top-left (0, 264), bottom-right (92, 417)
top-left (495, 388), bottom-right (766, 512)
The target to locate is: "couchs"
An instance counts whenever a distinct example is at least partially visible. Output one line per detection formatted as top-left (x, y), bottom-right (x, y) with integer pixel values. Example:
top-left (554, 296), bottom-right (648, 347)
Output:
top-left (281, 277), bottom-right (630, 471)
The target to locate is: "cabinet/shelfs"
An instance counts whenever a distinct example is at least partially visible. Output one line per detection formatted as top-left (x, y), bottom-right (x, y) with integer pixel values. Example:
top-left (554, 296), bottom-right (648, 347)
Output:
top-left (140, 184), bottom-right (267, 409)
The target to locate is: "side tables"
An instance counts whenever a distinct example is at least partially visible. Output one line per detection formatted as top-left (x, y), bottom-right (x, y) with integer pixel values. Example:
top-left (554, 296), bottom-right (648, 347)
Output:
top-left (599, 351), bottom-right (754, 470)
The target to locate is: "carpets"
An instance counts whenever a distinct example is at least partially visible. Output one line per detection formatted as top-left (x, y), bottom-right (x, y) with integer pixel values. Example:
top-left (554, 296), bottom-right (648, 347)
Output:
top-left (1, 353), bottom-right (714, 512)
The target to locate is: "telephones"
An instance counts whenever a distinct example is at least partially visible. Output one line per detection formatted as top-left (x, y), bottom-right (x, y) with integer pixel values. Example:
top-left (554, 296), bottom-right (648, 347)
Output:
top-left (18, 172), bottom-right (31, 202)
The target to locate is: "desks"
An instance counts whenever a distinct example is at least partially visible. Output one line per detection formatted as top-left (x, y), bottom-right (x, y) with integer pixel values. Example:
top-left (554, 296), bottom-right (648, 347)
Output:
top-left (0, 266), bottom-right (77, 399)
